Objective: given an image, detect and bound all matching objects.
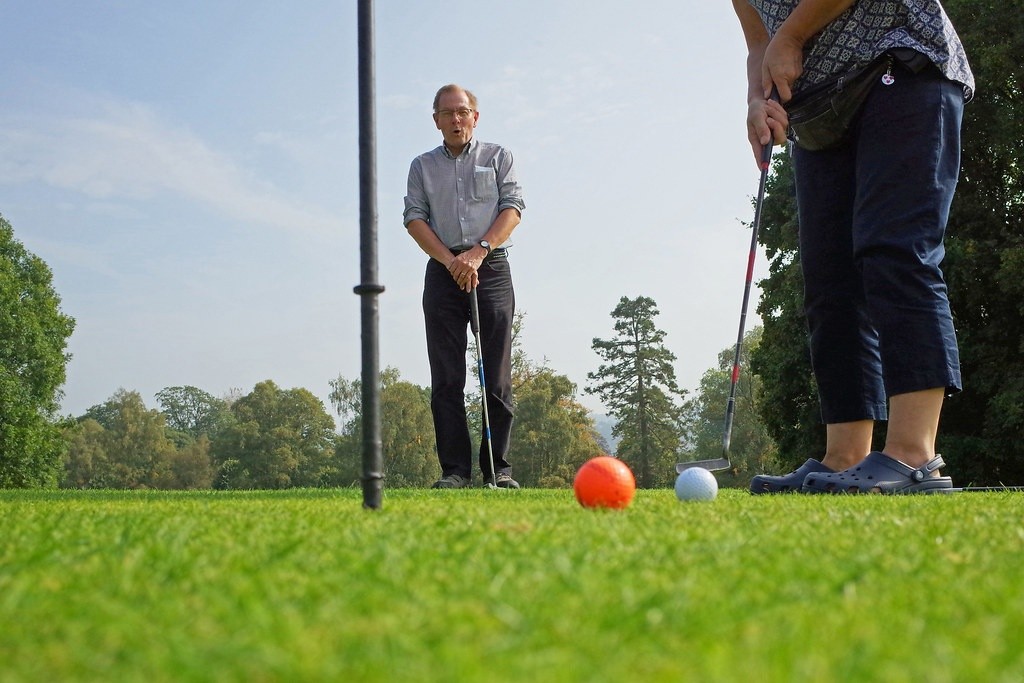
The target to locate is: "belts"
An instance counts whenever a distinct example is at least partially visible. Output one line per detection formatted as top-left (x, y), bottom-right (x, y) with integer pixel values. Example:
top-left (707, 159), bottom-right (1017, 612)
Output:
top-left (449, 249), bottom-right (507, 259)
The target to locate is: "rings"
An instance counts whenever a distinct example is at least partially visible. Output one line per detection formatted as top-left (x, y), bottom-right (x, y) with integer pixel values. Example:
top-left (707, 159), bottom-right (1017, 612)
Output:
top-left (461, 273), bottom-right (465, 276)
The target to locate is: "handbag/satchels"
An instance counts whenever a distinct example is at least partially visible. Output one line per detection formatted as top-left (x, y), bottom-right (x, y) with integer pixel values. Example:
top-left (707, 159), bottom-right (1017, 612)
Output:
top-left (781, 56), bottom-right (894, 153)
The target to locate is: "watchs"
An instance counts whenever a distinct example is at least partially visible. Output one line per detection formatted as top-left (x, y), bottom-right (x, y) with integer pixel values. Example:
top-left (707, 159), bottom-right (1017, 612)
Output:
top-left (477, 239), bottom-right (491, 256)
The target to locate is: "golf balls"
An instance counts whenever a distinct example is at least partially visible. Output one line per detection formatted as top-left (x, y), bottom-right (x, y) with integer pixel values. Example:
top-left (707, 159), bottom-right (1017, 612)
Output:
top-left (675, 466), bottom-right (718, 504)
top-left (573, 456), bottom-right (635, 510)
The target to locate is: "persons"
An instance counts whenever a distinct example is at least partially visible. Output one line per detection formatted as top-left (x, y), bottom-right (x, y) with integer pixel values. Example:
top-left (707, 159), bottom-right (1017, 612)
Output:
top-left (403, 85), bottom-right (523, 488)
top-left (732, 0), bottom-right (974, 493)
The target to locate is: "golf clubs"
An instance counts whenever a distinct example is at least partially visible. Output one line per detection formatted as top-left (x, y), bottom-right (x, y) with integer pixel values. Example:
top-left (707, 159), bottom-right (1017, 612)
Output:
top-left (674, 81), bottom-right (780, 476)
top-left (471, 287), bottom-right (497, 490)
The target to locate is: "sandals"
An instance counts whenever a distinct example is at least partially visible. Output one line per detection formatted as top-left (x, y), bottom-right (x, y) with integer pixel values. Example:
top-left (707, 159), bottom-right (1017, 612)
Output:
top-left (750, 458), bottom-right (837, 497)
top-left (802, 451), bottom-right (953, 496)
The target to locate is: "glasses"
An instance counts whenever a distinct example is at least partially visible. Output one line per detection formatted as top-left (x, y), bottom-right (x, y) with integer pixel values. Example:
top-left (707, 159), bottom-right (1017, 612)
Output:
top-left (435, 109), bottom-right (472, 119)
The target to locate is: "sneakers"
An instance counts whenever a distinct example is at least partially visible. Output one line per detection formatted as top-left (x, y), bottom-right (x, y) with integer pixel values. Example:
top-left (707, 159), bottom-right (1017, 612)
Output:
top-left (430, 474), bottom-right (473, 489)
top-left (483, 472), bottom-right (520, 490)
